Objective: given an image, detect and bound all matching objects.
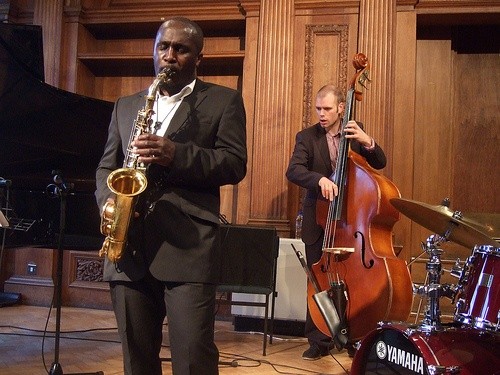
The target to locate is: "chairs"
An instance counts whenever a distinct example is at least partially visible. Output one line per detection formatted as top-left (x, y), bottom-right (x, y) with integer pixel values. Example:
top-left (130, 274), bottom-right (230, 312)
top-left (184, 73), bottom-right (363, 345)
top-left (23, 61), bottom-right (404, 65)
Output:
top-left (217, 224), bottom-right (280, 357)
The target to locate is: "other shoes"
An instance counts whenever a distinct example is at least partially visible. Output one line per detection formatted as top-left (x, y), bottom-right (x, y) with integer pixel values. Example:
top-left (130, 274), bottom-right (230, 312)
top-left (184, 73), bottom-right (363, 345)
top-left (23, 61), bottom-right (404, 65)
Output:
top-left (301, 344), bottom-right (332, 361)
top-left (347, 346), bottom-right (359, 358)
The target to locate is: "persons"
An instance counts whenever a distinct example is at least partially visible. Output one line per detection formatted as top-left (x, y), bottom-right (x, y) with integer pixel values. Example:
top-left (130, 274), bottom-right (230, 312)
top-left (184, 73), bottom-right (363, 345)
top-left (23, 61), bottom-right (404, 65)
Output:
top-left (95, 16), bottom-right (248, 375)
top-left (286, 85), bottom-right (387, 360)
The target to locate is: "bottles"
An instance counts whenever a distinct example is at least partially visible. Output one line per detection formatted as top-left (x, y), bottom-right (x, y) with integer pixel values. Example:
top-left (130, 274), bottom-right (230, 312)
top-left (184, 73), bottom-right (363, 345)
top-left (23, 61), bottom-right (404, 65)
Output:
top-left (296, 212), bottom-right (303, 239)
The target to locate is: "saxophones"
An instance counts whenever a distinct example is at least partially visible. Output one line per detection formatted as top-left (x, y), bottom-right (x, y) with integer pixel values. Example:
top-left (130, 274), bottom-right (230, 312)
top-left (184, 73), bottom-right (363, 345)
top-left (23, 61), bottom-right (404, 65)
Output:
top-left (99, 66), bottom-right (171, 262)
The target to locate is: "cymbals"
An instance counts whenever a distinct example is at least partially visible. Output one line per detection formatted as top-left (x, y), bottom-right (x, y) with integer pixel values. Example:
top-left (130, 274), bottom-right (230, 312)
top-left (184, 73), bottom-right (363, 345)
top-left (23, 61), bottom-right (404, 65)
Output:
top-left (390, 198), bottom-right (500, 250)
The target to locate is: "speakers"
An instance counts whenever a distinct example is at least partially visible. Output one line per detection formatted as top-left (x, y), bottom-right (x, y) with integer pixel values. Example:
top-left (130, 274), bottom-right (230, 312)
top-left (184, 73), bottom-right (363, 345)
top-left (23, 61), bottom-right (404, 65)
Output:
top-left (215, 223), bottom-right (277, 295)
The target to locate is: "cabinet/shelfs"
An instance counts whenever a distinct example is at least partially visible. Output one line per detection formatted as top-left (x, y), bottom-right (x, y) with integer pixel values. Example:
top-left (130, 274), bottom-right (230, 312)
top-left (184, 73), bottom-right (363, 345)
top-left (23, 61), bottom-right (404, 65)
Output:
top-left (231, 237), bottom-right (307, 338)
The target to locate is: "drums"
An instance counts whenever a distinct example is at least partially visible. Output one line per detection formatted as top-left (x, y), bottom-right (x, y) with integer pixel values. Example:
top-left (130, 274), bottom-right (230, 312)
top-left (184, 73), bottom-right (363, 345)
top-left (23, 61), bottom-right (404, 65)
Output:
top-left (453, 245), bottom-right (500, 332)
top-left (350, 317), bottom-right (500, 375)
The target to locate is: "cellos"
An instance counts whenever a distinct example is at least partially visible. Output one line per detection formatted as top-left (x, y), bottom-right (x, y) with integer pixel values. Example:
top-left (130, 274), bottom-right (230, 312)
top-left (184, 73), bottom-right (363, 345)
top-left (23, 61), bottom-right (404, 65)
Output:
top-left (307, 53), bottom-right (416, 342)
